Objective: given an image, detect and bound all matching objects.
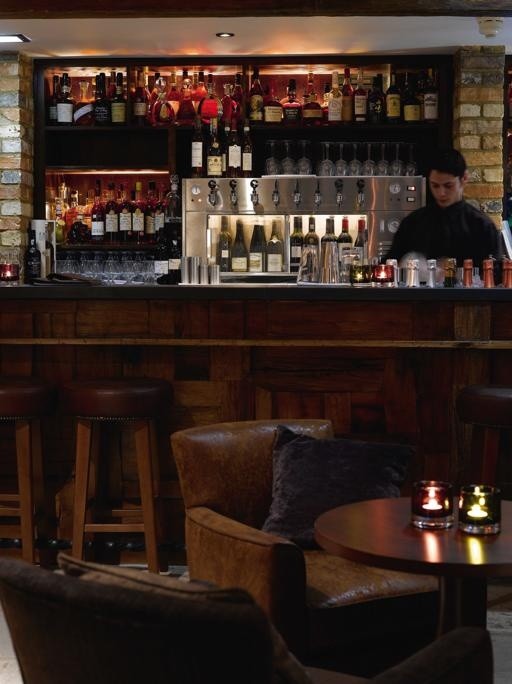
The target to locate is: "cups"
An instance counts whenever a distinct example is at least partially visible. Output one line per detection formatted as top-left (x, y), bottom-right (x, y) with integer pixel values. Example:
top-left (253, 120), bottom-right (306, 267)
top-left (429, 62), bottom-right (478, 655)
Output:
top-left (57, 249), bottom-right (155, 285)
top-left (372, 259), bottom-right (395, 287)
top-left (0, 260), bottom-right (20, 281)
top-left (458, 485), bottom-right (501, 536)
top-left (350, 259), bottom-right (372, 287)
top-left (410, 480), bottom-right (454, 529)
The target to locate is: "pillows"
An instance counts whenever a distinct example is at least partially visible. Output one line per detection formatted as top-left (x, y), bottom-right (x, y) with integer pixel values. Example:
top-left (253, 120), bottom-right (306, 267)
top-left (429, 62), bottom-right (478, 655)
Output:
top-left (259, 426), bottom-right (422, 550)
top-left (56, 549), bottom-right (253, 601)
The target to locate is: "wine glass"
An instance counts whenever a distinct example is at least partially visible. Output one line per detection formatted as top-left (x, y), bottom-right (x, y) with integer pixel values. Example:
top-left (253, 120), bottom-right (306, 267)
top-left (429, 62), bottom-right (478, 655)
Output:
top-left (263, 139), bottom-right (420, 177)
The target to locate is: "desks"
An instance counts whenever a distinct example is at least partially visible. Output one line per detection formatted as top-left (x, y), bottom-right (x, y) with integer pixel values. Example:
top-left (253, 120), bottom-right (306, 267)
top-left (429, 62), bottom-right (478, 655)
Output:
top-left (314, 492), bottom-right (512, 645)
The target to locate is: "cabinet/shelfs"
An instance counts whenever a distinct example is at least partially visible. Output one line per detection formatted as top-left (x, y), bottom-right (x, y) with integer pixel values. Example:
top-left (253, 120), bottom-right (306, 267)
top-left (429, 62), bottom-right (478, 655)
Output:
top-left (32, 54), bottom-right (454, 281)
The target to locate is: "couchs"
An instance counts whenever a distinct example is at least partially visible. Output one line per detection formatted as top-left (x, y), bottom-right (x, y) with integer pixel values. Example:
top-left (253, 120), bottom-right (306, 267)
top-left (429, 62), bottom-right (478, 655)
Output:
top-left (171, 419), bottom-right (487, 668)
top-left (0, 557), bottom-right (493, 684)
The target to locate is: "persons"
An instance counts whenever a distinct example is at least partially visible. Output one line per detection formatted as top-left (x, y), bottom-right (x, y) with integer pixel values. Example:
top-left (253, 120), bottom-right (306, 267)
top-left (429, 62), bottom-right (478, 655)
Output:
top-left (375, 148), bottom-right (505, 286)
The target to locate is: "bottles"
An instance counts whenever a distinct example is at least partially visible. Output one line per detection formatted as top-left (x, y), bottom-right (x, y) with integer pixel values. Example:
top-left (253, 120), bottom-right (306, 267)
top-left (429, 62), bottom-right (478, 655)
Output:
top-left (191, 113), bottom-right (257, 178)
top-left (321, 218), bottom-right (340, 268)
top-left (180, 256), bottom-right (220, 285)
top-left (338, 217), bottom-right (354, 265)
top-left (266, 220), bottom-right (284, 272)
top-left (24, 227), bottom-right (41, 284)
top-left (248, 225), bottom-right (268, 273)
top-left (290, 216), bottom-right (305, 273)
top-left (304, 217), bottom-right (319, 264)
top-left (154, 228), bottom-right (168, 285)
top-left (52, 179), bottom-right (181, 242)
top-left (216, 216), bottom-right (232, 272)
top-left (167, 229), bottom-right (181, 284)
top-left (353, 217), bottom-right (367, 264)
top-left (231, 219), bottom-right (249, 272)
top-left (296, 239), bottom-right (342, 282)
top-left (383, 259), bottom-right (511, 287)
top-left (46, 63), bottom-right (442, 126)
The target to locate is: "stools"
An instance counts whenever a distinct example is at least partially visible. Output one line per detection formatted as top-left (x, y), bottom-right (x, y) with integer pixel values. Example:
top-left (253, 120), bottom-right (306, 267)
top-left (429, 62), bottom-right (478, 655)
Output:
top-left (0, 378), bottom-right (54, 567)
top-left (67, 377), bottom-right (171, 574)
top-left (455, 385), bottom-right (511, 491)
